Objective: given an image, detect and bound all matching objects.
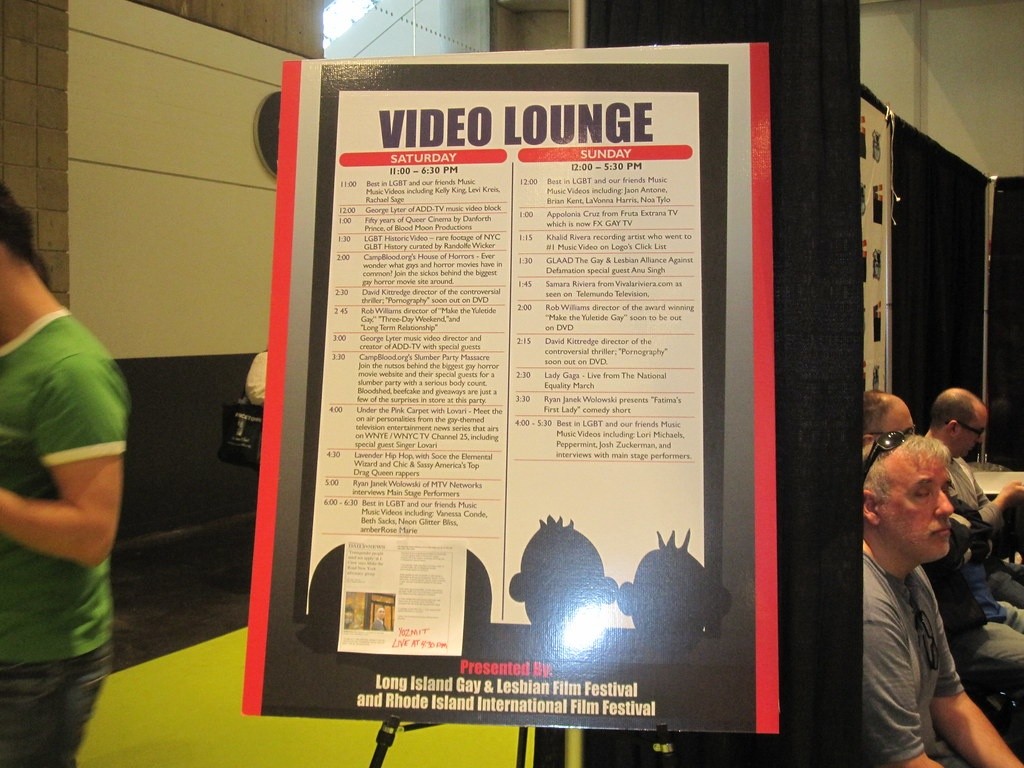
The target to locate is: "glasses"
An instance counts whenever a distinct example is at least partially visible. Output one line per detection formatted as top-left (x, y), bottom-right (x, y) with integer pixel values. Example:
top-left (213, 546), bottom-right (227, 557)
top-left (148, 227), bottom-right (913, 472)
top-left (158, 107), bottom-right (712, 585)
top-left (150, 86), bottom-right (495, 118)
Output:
top-left (870, 424), bottom-right (917, 440)
top-left (865, 430), bottom-right (906, 475)
top-left (944, 417), bottom-right (985, 439)
top-left (916, 609), bottom-right (940, 670)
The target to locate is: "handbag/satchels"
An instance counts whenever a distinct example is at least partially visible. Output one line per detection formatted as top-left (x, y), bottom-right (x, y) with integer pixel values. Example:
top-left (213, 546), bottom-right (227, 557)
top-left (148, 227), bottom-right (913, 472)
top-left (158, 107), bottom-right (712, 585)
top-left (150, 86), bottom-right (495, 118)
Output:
top-left (941, 596), bottom-right (988, 636)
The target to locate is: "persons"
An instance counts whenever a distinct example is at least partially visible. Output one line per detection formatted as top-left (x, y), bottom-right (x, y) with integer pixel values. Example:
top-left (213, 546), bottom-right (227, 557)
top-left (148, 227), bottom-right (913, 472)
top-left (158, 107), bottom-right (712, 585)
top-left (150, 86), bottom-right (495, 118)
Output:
top-left (863, 390), bottom-right (1024, 706)
top-left (862, 435), bottom-right (1024, 768)
top-left (1, 183), bottom-right (132, 768)
top-left (923, 388), bottom-right (1024, 609)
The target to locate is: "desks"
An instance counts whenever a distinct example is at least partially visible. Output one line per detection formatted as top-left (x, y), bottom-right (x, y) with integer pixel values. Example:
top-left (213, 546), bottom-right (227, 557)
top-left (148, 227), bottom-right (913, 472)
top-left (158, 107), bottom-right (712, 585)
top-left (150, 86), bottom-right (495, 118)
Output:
top-left (973, 471), bottom-right (1024, 557)
